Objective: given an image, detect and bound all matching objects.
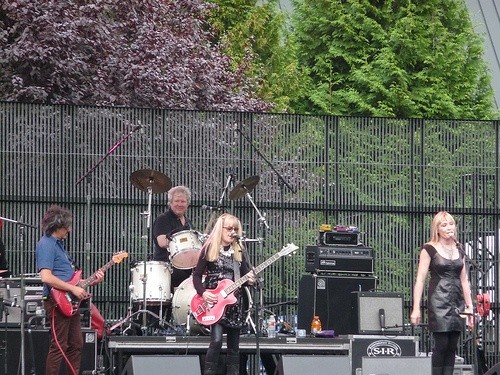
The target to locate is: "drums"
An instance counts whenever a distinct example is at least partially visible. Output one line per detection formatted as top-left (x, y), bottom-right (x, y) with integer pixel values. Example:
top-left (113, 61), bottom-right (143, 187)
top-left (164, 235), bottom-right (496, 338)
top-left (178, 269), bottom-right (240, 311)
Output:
top-left (171, 274), bottom-right (253, 336)
top-left (169, 230), bottom-right (204, 270)
top-left (130, 260), bottom-right (173, 307)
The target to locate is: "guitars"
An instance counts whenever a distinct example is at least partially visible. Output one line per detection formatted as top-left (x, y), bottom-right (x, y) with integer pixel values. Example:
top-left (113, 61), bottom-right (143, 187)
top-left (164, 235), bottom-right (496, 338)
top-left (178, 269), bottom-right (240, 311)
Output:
top-left (190, 242), bottom-right (300, 327)
top-left (50, 250), bottom-right (128, 318)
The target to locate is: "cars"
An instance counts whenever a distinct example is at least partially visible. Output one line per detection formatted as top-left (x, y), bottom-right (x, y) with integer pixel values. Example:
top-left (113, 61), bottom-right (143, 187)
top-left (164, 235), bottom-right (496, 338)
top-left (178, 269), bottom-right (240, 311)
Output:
top-left (466, 235), bottom-right (500, 375)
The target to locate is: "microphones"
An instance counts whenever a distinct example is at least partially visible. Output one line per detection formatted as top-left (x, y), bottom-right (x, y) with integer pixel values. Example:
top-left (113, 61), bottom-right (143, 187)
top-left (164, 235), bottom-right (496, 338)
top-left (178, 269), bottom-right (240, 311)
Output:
top-left (379, 309), bottom-right (385, 335)
top-left (448, 233), bottom-right (465, 249)
top-left (201, 205), bottom-right (224, 211)
top-left (229, 232), bottom-right (240, 239)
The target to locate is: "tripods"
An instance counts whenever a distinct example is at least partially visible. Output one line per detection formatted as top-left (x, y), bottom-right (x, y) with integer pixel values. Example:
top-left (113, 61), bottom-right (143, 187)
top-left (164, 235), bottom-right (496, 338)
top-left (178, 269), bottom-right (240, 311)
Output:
top-left (109, 186), bottom-right (183, 336)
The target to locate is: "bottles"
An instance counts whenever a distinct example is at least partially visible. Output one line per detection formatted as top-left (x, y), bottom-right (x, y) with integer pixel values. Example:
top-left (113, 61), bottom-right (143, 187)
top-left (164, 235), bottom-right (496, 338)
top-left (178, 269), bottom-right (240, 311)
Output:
top-left (267, 315), bottom-right (276, 339)
top-left (311, 315), bottom-right (322, 335)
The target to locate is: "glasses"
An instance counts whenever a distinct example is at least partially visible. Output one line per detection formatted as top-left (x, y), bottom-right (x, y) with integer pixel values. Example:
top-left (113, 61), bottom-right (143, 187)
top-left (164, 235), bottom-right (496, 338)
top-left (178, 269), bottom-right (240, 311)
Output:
top-left (222, 225), bottom-right (240, 233)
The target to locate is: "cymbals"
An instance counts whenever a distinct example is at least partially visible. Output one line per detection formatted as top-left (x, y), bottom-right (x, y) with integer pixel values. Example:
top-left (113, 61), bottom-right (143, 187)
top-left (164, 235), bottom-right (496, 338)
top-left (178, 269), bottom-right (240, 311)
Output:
top-left (130, 169), bottom-right (172, 194)
top-left (229, 175), bottom-right (260, 201)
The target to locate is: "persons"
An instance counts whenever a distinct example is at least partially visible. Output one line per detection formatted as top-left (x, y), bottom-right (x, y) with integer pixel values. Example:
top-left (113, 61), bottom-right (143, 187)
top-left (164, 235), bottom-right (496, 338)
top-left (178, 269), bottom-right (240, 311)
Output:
top-left (38, 206), bottom-right (105, 375)
top-left (152, 186), bottom-right (195, 288)
top-left (410, 211), bottom-right (473, 375)
top-left (193, 213), bottom-right (256, 375)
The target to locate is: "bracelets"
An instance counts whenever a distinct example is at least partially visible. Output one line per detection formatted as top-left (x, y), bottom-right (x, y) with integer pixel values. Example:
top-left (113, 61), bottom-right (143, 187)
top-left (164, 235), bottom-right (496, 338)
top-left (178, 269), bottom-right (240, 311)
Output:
top-left (466, 305), bottom-right (473, 308)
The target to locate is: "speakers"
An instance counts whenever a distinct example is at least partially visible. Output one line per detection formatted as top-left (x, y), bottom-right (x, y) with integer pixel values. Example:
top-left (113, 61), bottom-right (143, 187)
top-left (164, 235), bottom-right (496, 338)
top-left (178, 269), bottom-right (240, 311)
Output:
top-left (120, 355), bottom-right (201, 375)
top-left (483, 362), bottom-right (500, 375)
top-left (278, 355), bottom-right (352, 375)
top-left (297, 274), bottom-right (377, 336)
top-left (362, 356), bottom-right (431, 375)
